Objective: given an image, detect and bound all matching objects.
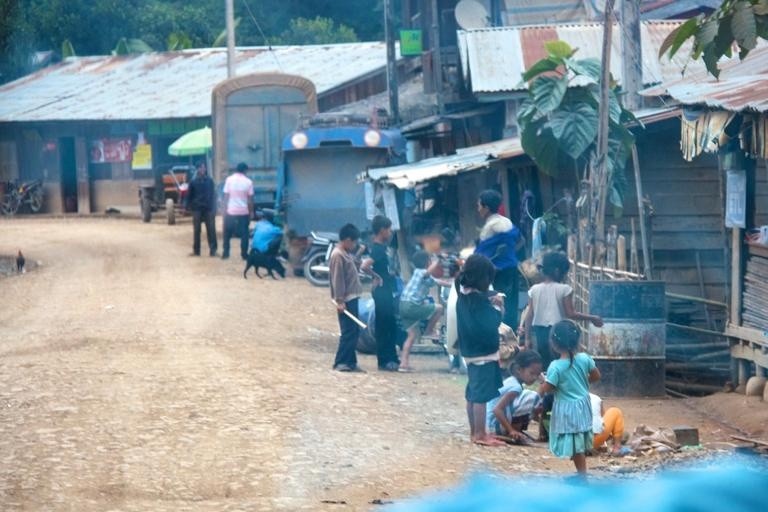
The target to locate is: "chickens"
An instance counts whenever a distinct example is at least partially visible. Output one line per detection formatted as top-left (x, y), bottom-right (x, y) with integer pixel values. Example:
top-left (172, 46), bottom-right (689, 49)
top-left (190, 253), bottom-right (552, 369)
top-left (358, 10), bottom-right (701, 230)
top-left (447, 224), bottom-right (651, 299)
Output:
top-left (16, 249), bottom-right (25, 272)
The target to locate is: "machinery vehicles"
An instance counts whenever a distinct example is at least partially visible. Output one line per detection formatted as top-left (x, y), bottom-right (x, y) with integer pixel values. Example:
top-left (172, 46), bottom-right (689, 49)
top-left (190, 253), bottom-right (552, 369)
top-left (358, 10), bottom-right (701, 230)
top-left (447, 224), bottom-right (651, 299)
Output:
top-left (137, 162), bottom-right (199, 224)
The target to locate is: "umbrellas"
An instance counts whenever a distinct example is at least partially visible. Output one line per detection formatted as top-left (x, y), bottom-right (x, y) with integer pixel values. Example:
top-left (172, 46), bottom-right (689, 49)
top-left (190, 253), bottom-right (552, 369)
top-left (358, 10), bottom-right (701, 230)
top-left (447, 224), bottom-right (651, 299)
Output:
top-left (168, 124), bottom-right (213, 177)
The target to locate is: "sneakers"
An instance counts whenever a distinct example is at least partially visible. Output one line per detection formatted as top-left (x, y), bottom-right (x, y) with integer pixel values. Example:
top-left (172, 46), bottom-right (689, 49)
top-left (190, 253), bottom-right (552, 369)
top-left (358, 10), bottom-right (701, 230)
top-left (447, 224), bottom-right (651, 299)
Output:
top-left (337, 361), bottom-right (415, 372)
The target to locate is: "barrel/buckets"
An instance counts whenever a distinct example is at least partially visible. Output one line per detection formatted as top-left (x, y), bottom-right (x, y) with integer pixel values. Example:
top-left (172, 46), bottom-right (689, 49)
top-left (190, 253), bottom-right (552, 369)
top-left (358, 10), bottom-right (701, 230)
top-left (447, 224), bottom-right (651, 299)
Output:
top-left (357, 299), bottom-right (375, 352)
top-left (587, 276), bottom-right (667, 398)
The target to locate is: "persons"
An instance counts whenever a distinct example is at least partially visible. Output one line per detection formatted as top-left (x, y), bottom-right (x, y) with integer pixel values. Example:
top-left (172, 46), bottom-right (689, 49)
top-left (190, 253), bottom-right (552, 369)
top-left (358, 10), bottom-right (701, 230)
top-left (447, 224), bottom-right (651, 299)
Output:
top-left (472, 192), bottom-right (526, 336)
top-left (588, 390), bottom-right (632, 458)
top-left (519, 252), bottom-right (602, 373)
top-left (329, 223), bottom-right (365, 373)
top-left (251, 210), bottom-right (285, 256)
top-left (397, 252), bottom-right (452, 371)
top-left (221, 163), bottom-right (255, 259)
top-left (541, 319), bottom-right (601, 472)
top-left (184, 159), bottom-right (218, 255)
top-left (486, 352), bottom-right (540, 445)
top-left (455, 253), bottom-right (505, 447)
top-left (537, 373), bottom-right (555, 444)
top-left (361, 215), bottom-right (401, 371)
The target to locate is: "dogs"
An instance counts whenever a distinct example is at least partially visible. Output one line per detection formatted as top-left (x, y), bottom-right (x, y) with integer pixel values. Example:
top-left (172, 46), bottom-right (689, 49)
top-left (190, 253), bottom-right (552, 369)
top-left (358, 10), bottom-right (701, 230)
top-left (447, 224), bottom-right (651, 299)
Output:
top-left (241, 250), bottom-right (286, 281)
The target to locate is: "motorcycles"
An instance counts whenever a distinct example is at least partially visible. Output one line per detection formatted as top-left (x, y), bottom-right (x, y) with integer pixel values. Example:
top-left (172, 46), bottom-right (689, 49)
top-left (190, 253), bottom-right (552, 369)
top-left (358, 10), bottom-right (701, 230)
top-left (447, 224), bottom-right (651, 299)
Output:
top-left (299, 229), bottom-right (401, 286)
top-left (5, 177), bottom-right (45, 214)
top-left (354, 228), bottom-right (466, 355)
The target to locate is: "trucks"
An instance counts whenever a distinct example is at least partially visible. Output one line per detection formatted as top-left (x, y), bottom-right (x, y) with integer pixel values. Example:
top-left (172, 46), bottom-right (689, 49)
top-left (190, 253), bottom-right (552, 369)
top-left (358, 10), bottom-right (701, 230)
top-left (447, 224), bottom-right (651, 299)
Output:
top-left (204, 73), bottom-right (318, 215)
top-left (273, 125), bottom-right (408, 277)
